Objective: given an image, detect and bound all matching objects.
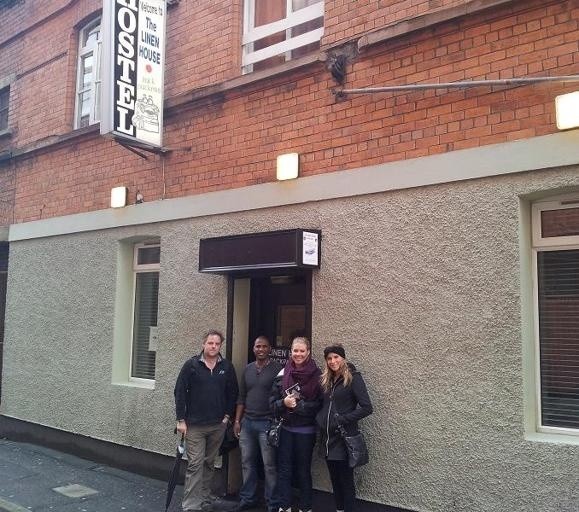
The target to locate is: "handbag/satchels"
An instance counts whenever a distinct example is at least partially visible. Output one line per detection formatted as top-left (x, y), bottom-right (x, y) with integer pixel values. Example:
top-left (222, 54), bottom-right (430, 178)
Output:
top-left (344, 431), bottom-right (370, 470)
top-left (266, 402), bottom-right (283, 447)
top-left (219, 416), bottom-right (238, 456)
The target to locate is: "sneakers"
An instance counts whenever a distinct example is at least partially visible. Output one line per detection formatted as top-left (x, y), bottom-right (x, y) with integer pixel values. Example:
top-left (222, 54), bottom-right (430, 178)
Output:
top-left (232, 501), bottom-right (251, 511)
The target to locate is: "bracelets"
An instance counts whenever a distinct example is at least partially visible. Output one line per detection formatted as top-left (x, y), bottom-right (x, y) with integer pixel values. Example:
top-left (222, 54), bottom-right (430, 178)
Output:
top-left (234, 420), bottom-right (239, 423)
top-left (223, 415), bottom-right (230, 420)
top-left (178, 419), bottom-right (184, 422)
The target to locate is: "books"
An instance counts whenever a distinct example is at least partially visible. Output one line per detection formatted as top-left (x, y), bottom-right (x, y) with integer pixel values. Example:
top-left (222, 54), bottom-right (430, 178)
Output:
top-left (284, 382), bottom-right (305, 404)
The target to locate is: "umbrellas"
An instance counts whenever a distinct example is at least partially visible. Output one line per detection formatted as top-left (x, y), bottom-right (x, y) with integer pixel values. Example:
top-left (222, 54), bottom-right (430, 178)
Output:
top-left (164, 427), bottom-right (185, 512)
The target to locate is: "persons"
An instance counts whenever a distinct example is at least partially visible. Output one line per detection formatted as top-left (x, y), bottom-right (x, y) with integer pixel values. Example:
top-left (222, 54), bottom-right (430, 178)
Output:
top-left (268, 335), bottom-right (324, 512)
top-left (171, 329), bottom-right (238, 512)
top-left (232, 335), bottom-right (284, 512)
top-left (315, 344), bottom-right (372, 512)
